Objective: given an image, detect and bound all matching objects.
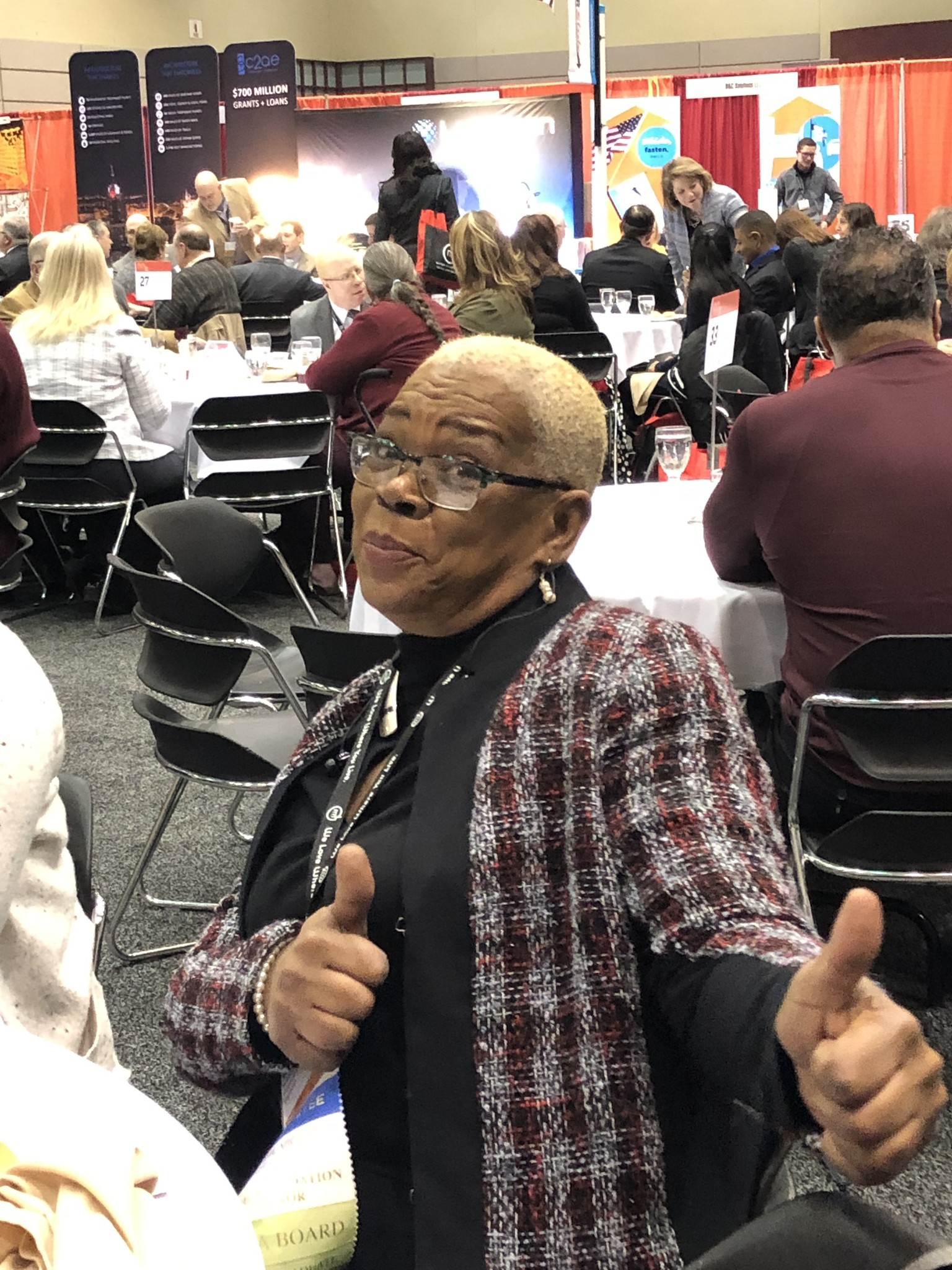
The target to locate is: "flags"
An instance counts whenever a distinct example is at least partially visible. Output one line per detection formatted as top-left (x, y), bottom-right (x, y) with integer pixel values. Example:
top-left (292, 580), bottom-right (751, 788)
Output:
top-left (591, 113), bottom-right (643, 174)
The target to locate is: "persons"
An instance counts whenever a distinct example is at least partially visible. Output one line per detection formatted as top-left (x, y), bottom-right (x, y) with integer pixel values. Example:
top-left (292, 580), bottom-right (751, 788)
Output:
top-left (306, 239), bottom-right (464, 592)
top-left (836, 202), bottom-right (877, 238)
top-left (448, 210), bottom-right (535, 342)
top-left (0, 321), bottom-right (40, 578)
top-left (704, 226), bottom-right (952, 837)
top-left (0, 171), bottom-right (378, 351)
top-left (682, 209), bottom-right (795, 317)
top-left (660, 157), bottom-right (749, 301)
top-left (142, 224), bottom-right (242, 340)
top-left (917, 205), bottom-right (952, 359)
top-left (510, 204), bottom-right (613, 381)
top-left (619, 222), bottom-right (759, 474)
top-left (374, 130), bottom-right (461, 296)
top-left (10, 232), bottom-right (184, 614)
top-left (773, 209), bottom-right (840, 324)
top-left (775, 137), bottom-right (842, 228)
top-left (580, 203), bottom-right (678, 314)
top-left (159, 333), bottom-right (952, 1270)
top-left (0, 616), bottom-right (265, 1270)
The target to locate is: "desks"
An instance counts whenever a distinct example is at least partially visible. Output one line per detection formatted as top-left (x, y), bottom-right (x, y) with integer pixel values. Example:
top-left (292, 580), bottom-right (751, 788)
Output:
top-left (156, 349), bottom-right (324, 513)
top-left (590, 310), bottom-right (687, 387)
top-left (350, 472), bottom-right (786, 724)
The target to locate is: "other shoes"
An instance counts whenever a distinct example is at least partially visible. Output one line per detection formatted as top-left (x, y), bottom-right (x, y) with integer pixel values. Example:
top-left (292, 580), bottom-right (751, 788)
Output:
top-left (82, 580), bottom-right (130, 617)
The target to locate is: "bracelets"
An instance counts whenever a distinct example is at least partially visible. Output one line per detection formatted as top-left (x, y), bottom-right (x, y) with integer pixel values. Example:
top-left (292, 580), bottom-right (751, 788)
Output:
top-left (252, 936), bottom-right (295, 1033)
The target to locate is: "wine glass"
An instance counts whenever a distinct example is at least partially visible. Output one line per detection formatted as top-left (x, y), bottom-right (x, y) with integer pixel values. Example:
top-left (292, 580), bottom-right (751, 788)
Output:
top-left (615, 290), bottom-right (632, 316)
top-left (656, 425), bottom-right (692, 481)
top-left (599, 289), bottom-right (616, 314)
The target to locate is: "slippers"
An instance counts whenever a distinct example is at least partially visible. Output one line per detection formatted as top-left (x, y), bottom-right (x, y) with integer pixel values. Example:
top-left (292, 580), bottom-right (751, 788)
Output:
top-left (298, 577), bottom-right (343, 595)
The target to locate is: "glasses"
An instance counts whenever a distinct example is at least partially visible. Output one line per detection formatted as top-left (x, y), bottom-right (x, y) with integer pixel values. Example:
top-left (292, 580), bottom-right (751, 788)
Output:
top-left (347, 430), bottom-right (571, 512)
top-left (326, 267), bottom-right (360, 281)
top-left (799, 150), bottom-right (816, 158)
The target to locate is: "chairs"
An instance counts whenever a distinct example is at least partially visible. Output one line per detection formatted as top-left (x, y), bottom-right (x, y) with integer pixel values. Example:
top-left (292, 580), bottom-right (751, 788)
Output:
top-left (780, 629), bottom-right (952, 948)
top-left (287, 623), bottom-right (404, 734)
top-left (0, 436), bottom-right (42, 620)
top-left (182, 389), bottom-right (352, 619)
top-left (128, 490), bottom-right (336, 838)
top-left (104, 554), bottom-right (309, 960)
top-left (23, 390), bottom-right (150, 642)
top-left (537, 327), bottom-right (620, 483)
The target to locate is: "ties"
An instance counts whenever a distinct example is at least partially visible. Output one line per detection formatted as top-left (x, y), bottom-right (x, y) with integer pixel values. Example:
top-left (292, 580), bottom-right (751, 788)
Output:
top-left (347, 309), bottom-right (359, 318)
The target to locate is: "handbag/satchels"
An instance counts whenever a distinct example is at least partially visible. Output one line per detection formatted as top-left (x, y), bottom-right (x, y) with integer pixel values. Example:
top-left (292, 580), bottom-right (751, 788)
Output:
top-left (415, 175), bottom-right (460, 290)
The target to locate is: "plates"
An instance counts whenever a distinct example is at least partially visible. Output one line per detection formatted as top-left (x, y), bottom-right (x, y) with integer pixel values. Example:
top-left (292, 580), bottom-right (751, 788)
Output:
top-left (651, 314), bottom-right (687, 322)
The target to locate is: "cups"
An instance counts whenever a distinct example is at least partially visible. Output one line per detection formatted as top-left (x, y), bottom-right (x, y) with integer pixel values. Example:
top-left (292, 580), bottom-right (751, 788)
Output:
top-left (291, 336), bottom-right (322, 376)
top-left (638, 295), bottom-right (655, 318)
top-left (245, 332), bottom-right (273, 373)
